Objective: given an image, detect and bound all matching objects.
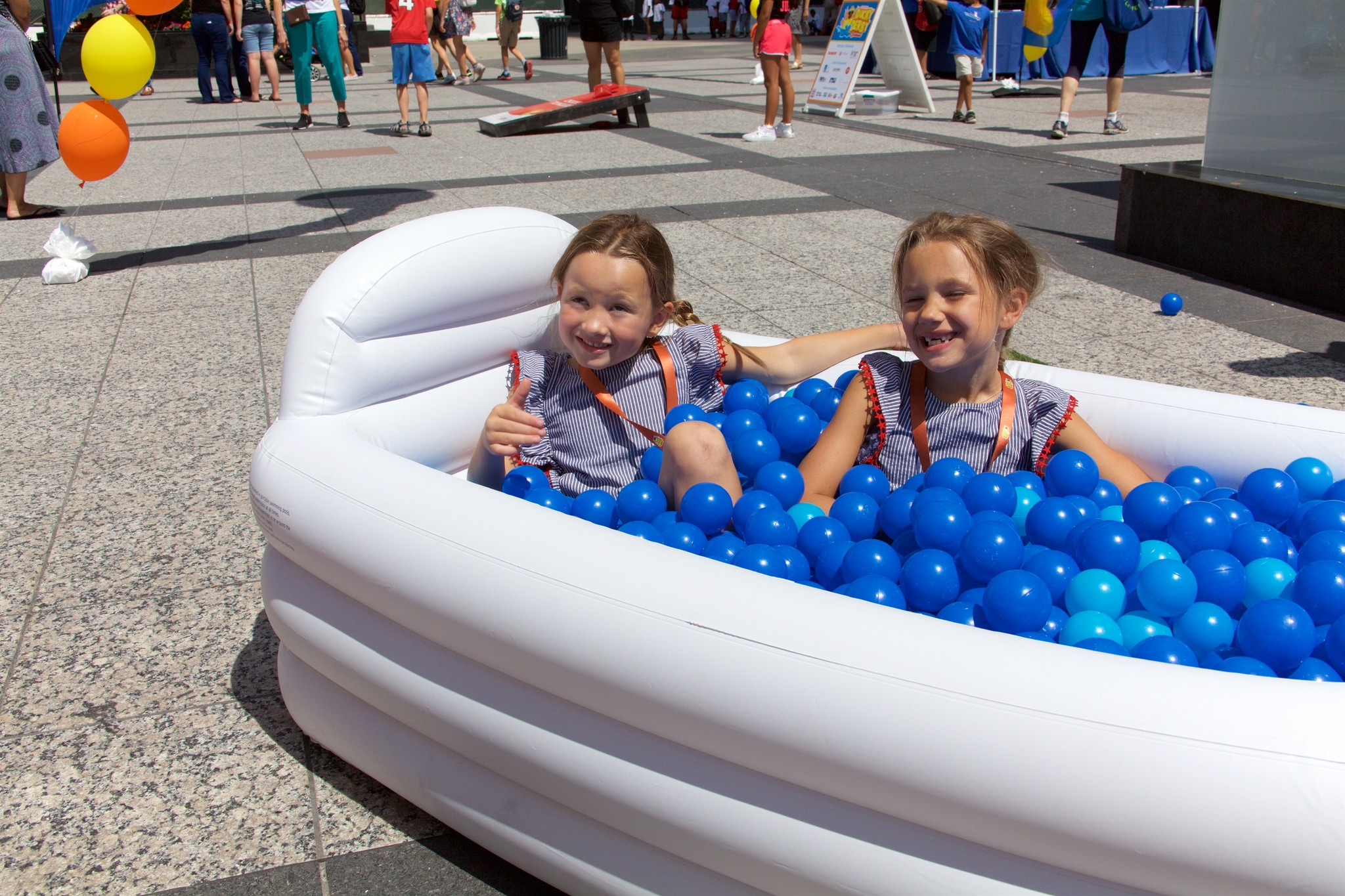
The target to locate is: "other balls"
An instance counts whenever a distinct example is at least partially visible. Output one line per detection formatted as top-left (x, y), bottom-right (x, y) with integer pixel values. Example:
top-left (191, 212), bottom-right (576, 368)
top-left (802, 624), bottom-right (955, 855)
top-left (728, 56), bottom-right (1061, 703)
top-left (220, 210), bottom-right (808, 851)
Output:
top-left (1160, 293), bottom-right (1183, 316)
top-left (502, 369), bottom-right (1345, 683)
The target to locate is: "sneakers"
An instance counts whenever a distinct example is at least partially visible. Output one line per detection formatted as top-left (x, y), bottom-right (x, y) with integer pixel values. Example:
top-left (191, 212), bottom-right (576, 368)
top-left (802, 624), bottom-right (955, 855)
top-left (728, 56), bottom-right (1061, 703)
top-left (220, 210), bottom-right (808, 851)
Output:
top-left (497, 71), bottom-right (512, 80)
top-left (293, 113), bottom-right (314, 130)
top-left (337, 110), bottom-right (351, 128)
top-left (523, 60), bottom-right (532, 80)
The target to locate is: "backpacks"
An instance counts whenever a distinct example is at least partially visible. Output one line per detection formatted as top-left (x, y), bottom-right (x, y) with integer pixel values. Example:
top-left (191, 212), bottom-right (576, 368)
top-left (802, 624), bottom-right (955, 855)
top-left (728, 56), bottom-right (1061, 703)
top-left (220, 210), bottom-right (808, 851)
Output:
top-left (458, 0), bottom-right (477, 7)
top-left (346, 0), bottom-right (366, 14)
top-left (501, 0), bottom-right (523, 23)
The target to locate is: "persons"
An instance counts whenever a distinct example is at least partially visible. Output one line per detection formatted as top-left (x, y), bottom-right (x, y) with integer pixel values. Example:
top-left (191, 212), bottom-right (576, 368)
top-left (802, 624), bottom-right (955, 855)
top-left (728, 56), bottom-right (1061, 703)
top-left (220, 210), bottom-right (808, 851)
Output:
top-left (273, 0), bottom-right (351, 129)
top-left (385, 0), bottom-right (434, 136)
top-left (466, 211), bottom-right (910, 507)
top-left (616, 0), bottom-right (693, 41)
top-left (580, 0), bottom-right (624, 116)
top-left (741, 0), bottom-right (802, 143)
top-left (901, 0), bottom-right (943, 79)
top-left (706, 0), bottom-right (752, 39)
top-left (795, 210), bottom-right (1153, 520)
top-left (429, 0), bottom-right (486, 86)
top-left (188, 0), bottom-right (243, 103)
top-left (321, 0), bottom-right (364, 80)
top-left (1051, 0), bottom-right (1130, 138)
top-left (926, 0), bottom-right (992, 123)
top-left (860, 47), bottom-right (882, 75)
top-left (226, 0), bottom-right (288, 101)
top-left (494, 0), bottom-right (533, 81)
top-left (785, 0), bottom-right (844, 70)
top-left (89, 0), bottom-right (155, 95)
top-left (0, 0), bottom-right (66, 221)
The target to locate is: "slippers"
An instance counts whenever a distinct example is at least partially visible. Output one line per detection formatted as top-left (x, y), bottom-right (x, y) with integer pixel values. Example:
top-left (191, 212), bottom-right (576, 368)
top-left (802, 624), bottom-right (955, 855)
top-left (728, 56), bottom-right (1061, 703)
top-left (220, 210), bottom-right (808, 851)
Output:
top-left (269, 96), bottom-right (282, 101)
top-left (222, 97), bottom-right (243, 103)
top-left (1, 203), bottom-right (60, 220)
top-left (249, 97), bottom-right (260, 102)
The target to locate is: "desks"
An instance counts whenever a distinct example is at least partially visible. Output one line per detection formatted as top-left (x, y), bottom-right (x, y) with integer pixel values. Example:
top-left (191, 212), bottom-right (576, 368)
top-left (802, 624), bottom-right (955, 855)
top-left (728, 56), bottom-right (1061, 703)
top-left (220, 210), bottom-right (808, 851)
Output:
top-left (927, 6), bottom-right (1216, 80)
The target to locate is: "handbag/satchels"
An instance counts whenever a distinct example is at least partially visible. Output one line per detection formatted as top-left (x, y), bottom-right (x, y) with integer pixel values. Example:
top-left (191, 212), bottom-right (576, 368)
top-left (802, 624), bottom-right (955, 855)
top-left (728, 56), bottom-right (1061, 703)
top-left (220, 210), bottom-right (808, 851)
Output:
top-left (924, 1), bottom-right (943, 24)
top-left (286, 4), bottom-right (310, 27)
top-left (30, 39), bottom-right (62, 75)
top-left (916, 0), bottom-right (939, 31)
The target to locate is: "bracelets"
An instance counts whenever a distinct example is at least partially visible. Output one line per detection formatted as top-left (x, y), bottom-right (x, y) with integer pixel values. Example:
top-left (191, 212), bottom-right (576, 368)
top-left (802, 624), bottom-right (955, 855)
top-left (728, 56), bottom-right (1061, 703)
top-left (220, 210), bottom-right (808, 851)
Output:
top-left (112, 8), bottom-right (115, 14)
top-left (338, 24), bottom-right (346, 28)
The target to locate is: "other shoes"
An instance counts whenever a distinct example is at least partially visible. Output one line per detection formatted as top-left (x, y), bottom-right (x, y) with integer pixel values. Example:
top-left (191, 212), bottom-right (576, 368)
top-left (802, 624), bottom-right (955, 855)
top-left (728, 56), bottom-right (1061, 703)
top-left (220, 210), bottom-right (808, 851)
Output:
top-left (442, 74), bottom-right (456, 85)
top-left (742, 123), bottom-right (797, 142)
top-left (240, 93), bottom-right (266, 99)
top-left (1103, 118), bottom-right (1130, 134)
top-left (343, 74), bottom-right (358, 81)
top-left (435, 70), bottom-right (444, 80)
top-left (790, 62), bottom-right (803, 69)
top-left (1051, 120), bottom-right (1070, 138)
top-left (390, 120), bottom-right (410, 133)
top-left (452, 75), bottom-right (471, 85)
top-left (466, 68), bottom-right (472, 76)
top-left (418, 122), bottom-right (432, 135)
top-left (923, 70), bottom-right (941, 80)
top-left (953, 110), bottom-right (976, 122)
top-left (473, 62), bottom-right (486, 84)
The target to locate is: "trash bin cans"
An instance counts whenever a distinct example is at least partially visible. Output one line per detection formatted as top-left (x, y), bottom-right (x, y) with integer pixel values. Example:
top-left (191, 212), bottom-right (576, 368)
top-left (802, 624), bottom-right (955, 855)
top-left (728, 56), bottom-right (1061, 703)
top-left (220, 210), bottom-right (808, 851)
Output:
top-left (534, 15), bottom-right (572, 59)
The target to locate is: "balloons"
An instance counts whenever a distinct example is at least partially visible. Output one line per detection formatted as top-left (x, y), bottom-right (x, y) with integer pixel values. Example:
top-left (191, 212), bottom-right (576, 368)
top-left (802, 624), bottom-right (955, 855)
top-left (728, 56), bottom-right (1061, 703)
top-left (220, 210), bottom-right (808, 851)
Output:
top-left (749, 0), bottom-right (760, 41)
top-left (56, 0), bottom-right (188, 181)
top-left (1023, 8), bottom-right (1053, 63)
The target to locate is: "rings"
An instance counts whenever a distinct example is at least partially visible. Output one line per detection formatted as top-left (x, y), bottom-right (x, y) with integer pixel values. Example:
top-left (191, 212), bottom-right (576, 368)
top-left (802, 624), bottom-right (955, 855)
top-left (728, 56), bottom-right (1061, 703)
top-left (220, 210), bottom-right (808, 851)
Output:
top-left (277, 47), bottom-right (281, 50)
